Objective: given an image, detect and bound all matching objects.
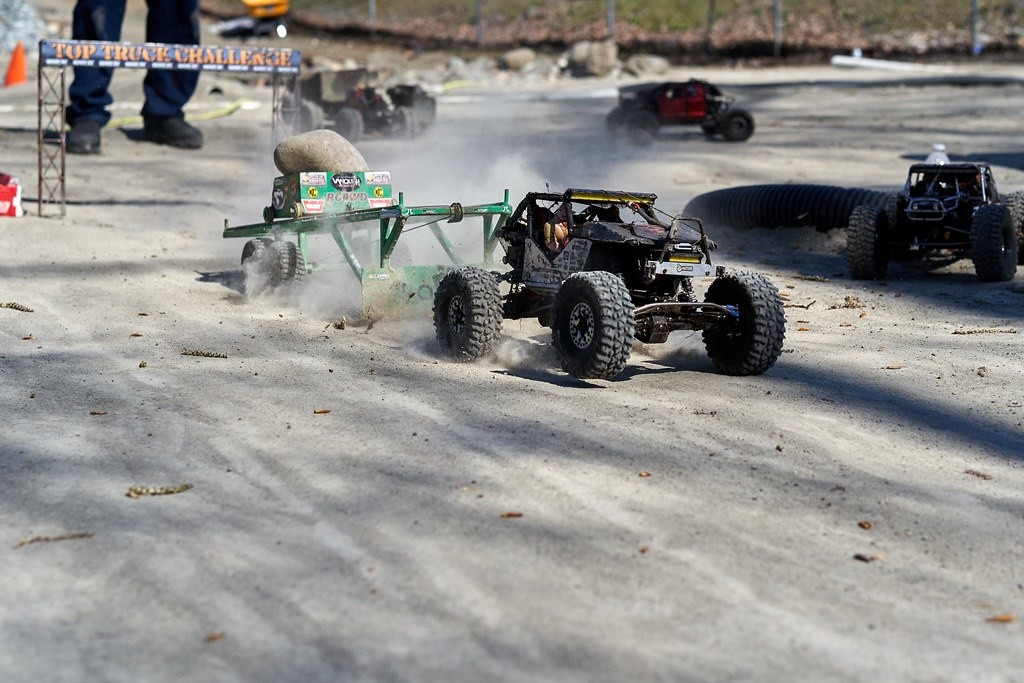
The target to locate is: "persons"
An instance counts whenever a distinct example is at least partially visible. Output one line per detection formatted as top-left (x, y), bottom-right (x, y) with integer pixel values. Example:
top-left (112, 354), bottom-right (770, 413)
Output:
top-left (918, 173), bottom-right (941, 190)
top-left (544, 203), bottom-right (572, 252)
top-left (968, 173), bottom-right (990, 197)
top-left (66, 0), bottom-right (203, 153)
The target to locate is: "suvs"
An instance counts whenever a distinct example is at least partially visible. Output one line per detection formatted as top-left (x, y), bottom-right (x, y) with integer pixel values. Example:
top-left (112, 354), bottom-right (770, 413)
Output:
top-left (433, 182), bottom-right (786, 378)
top-left (846, 161), bottom-right (1024, 281)
top-left (335, 82), bottom-right (420, 143)
top-left (605, 79), bottom-right (754, 142)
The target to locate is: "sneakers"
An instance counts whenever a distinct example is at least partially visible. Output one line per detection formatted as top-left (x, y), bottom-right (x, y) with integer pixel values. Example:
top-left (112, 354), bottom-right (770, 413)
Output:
top-left (67, 121), bottom-right (100, 153)
top-left (144, 115), bottom-right (202, 148)
